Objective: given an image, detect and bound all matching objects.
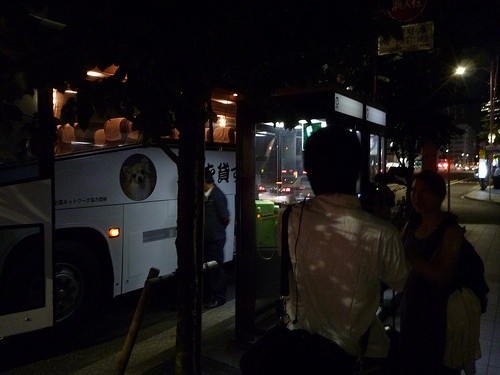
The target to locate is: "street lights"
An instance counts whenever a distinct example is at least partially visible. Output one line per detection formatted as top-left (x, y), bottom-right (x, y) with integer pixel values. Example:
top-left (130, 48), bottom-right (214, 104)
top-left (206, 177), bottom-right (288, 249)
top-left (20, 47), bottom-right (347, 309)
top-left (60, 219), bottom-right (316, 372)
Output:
top-left (453, 60), bottom-right (495, 201)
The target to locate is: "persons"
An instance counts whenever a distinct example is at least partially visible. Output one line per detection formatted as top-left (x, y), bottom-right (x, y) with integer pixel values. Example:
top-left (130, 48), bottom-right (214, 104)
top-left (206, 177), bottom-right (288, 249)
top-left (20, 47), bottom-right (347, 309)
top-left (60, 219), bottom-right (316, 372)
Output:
top-left (275, 125), bottom-right (489, 375)
top-left (203, 169), bottom-right (229, 308)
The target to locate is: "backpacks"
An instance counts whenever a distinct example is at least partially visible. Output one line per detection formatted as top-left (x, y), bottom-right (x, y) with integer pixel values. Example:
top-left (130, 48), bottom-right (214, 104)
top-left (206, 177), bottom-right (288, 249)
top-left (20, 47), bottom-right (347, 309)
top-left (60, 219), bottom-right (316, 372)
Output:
top-left (438, 214), bottom-right (488, 316)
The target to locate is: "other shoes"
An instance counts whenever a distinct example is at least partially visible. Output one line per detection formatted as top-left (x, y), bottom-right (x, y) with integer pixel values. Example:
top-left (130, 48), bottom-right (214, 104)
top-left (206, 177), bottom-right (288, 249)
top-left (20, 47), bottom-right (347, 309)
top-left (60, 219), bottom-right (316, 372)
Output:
top-left (204, 295), bottom-right (226, 309)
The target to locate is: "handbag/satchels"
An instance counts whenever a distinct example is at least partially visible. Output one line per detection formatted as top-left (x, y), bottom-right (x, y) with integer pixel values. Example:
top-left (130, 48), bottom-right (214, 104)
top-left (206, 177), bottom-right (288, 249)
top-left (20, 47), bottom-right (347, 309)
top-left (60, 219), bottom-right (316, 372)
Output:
top-left (240, 325), bottom-right (382, 375)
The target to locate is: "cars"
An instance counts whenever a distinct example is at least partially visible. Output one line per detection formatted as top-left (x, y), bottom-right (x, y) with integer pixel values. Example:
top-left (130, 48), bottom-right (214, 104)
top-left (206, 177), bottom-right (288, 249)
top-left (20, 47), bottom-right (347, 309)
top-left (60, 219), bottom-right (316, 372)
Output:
top-left (257, 175), bottom-right (316, 206)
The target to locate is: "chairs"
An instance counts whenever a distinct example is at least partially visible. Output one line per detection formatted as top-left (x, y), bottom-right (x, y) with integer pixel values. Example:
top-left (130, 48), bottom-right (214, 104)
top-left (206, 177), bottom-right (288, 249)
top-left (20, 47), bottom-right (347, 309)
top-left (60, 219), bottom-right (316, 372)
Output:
top-left (55, 117), bottom-right (237, 155)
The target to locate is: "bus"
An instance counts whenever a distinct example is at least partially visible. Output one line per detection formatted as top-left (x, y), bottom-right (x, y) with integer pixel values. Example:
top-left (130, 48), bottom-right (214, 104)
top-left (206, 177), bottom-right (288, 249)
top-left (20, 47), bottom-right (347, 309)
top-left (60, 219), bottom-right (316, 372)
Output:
top-left (0, 9), bottom-right (241, 359)
